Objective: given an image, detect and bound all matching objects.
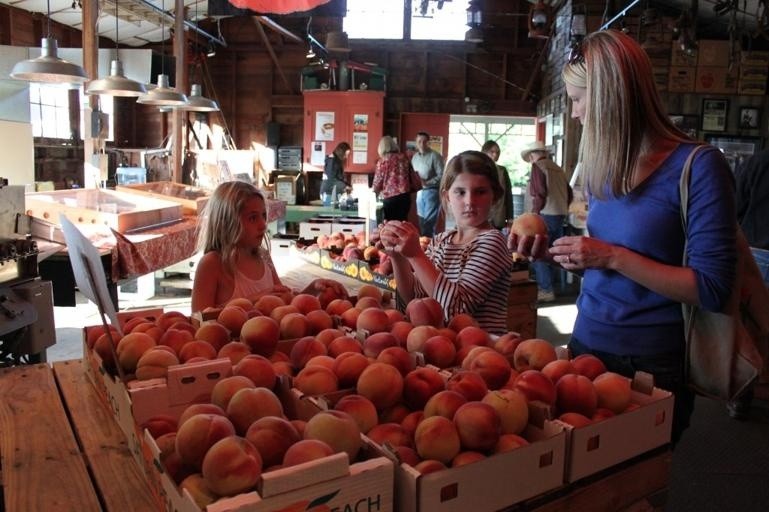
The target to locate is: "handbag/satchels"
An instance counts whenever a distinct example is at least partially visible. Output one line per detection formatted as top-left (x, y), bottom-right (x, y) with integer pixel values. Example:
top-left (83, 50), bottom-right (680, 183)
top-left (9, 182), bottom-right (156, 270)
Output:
top-left (682, 229), bottom-right (768, 402)
top-left (410, 170), bottom-right (422, 193)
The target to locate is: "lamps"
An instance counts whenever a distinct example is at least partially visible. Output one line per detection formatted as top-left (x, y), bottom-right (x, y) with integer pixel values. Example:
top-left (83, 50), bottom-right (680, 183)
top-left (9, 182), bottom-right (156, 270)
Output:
top-left (9, 0), bottom-right (89, 82)
top-left (136, 0), bottom-right (189, 106)
top-left (86, 0), bottom-right (149, 96)
top-left (175, 0), bottom-right (220, 112)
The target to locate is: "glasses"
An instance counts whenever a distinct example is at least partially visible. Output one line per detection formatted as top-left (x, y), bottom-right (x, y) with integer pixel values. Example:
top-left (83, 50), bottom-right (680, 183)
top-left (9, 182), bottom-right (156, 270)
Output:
top-left (568, 33), bottom-right (585, 64)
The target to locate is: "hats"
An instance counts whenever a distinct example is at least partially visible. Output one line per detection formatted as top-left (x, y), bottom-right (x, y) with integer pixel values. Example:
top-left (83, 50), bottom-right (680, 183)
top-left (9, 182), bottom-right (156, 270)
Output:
top-left (521, 140), bottom-right (553, 162)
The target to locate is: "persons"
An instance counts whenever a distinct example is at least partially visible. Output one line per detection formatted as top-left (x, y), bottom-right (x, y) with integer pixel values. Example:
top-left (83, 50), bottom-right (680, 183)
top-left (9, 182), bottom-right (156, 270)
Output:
top-left (481, 141), bottom-right (513, 230)
top-left (506, 30), bottom-right (737, 512)
top-left (192, 182), bottom-right (350, 314)
top-left (411, 133), bottom-right (444, 238)
top-left (372, 151), bottom-right (513, 336)
top-left (373, 136), bottom-right (411, 221)
top-left (320, 142), bottom-right (352, 209)
top-left (522, 143), bottom-right (573, 302)
top-left (725, 149), bottom-right (769, 420)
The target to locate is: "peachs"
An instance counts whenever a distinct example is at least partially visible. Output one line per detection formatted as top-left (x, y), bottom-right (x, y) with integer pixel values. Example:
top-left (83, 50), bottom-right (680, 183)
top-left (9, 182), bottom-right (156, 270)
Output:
top-left (306, 210), bottom-right (550, 282)
top-left (90, 283), bottom-right (646, 511)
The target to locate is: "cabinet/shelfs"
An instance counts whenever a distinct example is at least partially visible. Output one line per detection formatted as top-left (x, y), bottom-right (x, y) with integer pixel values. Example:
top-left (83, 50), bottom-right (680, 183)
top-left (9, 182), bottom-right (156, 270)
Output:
top-left (304, 91), bottom-right (385, 173)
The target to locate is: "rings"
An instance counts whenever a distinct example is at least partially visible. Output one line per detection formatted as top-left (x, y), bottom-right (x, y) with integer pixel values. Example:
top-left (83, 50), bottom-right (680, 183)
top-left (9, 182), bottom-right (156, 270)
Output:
top-left (567, 254), bottom-right (571, 264)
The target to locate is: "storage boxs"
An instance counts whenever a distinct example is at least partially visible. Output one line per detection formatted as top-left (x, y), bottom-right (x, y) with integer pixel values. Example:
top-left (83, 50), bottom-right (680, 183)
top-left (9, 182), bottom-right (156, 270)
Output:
top-left (82, 300), bottom-right (676, 511)
top-left (621, 16), bottom-right (768, 96)
